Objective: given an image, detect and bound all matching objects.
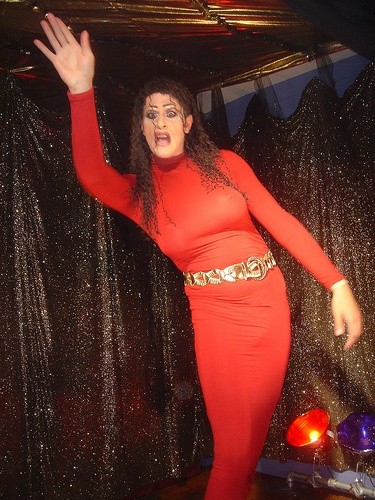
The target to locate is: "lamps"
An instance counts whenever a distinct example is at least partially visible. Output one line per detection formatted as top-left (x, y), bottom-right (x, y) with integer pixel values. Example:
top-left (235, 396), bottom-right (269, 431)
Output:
top-left (334, 412), bottom-right (375, 489)
top-left (284, 408), bottom-right (332, 481)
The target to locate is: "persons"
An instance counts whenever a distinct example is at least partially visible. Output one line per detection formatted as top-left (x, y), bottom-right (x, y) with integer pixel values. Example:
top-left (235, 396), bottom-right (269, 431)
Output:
top-left (34, 12), bottom-right (364, 500)
